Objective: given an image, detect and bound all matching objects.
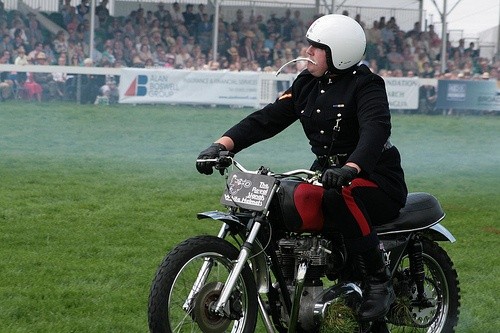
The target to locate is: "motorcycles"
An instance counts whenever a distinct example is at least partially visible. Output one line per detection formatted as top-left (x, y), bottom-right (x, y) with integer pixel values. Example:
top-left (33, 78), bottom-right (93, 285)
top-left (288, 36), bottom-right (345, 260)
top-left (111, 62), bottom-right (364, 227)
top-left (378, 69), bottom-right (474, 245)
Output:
top-left (146, 150), bottom-right (462, 332)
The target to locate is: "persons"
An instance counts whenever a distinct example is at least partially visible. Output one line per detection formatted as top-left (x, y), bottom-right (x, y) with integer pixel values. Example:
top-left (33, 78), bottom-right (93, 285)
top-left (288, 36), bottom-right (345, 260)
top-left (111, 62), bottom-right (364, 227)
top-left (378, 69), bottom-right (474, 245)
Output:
top-left (0, 0), bottom-right (500, 115)
top-left (196, 14), bottom-right (408, 322)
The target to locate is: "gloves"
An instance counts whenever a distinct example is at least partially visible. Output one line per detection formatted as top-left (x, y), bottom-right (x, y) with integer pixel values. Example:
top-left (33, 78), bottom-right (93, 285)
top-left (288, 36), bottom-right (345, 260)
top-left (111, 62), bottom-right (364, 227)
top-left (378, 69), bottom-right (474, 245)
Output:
top-left (194, 142), bottom-right (226, 176)
top-left (321, 164), bottom-right (357, 191)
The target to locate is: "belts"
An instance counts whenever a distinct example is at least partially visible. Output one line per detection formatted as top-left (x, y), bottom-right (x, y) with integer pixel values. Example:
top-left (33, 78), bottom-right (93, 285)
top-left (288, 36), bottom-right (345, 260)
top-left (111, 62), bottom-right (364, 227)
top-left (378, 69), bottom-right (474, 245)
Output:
top-left (317, 138), bottom-right (394, 165)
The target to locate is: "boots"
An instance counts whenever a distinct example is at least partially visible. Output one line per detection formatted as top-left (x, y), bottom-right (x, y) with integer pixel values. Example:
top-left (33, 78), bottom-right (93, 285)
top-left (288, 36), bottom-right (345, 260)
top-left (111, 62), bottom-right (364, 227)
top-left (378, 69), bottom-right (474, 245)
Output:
top-left (356, 237), bottom-right (396, 321)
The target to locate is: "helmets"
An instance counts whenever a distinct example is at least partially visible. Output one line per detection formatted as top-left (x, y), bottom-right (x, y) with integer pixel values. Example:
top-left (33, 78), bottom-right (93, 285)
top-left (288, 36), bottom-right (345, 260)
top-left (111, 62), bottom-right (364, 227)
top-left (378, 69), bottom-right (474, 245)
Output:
top-left (305, 13), bottom-right (367, 71)
